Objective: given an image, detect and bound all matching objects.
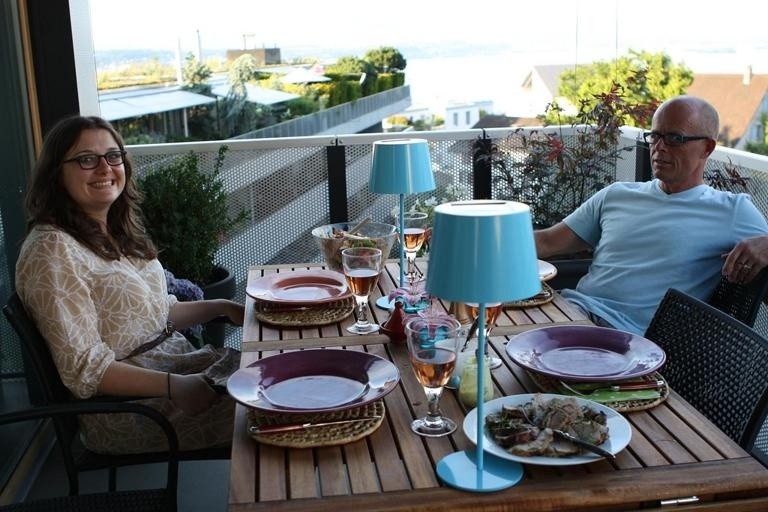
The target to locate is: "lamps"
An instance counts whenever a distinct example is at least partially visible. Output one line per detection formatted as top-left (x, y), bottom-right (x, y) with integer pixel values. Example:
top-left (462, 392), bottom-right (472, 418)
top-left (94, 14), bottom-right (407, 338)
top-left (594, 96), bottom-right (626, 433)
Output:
top-left (425, 192), bottom-right (542, 498)
top-left (371, 136), bottom-right (437, 309)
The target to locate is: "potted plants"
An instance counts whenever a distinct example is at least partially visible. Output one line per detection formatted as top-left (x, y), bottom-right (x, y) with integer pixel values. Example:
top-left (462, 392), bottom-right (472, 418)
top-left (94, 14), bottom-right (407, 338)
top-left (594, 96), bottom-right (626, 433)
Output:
top-left (132, 139), bottom-right (242, 345)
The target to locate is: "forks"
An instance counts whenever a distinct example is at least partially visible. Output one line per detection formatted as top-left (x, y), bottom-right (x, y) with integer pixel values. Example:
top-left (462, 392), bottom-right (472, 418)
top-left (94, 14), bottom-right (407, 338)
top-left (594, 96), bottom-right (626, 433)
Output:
top-left (560, 376), bottom-right (665, 396)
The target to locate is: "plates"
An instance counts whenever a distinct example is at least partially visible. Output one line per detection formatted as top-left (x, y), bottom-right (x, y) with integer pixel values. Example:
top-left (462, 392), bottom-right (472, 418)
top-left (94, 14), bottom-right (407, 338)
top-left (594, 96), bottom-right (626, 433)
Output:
top-left (503, 257), bottom-right (559, 312)
top-left (463, 393), bottom-right (632, 466)
top-left (246, 269), bottom-right (353, 305)
top-left (227, 348), bottom-right (401, 413)
top-left (506, 324), bottom-right (666, 383)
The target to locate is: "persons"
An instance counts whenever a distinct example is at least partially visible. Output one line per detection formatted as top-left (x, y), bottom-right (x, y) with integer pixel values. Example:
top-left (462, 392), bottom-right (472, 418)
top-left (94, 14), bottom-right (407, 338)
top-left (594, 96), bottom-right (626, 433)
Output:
top-left (15, 116), bottom-right (245, 455)
top-left (533, 96), bottom-right (768, 338)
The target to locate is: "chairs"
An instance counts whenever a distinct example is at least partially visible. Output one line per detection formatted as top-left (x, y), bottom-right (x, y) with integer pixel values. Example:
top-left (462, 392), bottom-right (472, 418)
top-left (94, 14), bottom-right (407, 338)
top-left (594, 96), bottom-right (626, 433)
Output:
top-left (631, 284), bottom-right (768, 451)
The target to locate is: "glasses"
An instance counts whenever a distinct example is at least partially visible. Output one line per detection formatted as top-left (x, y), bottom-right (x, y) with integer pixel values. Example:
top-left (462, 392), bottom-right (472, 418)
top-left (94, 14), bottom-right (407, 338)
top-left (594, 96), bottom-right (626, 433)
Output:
top-left (63, 151), bottom-right (128, 170)
top-left (644, 133), bottom-right (709, 147)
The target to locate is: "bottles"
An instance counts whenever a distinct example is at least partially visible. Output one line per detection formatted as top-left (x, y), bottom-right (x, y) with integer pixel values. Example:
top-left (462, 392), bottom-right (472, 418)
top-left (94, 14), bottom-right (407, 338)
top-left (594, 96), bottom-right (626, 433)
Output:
top-left (458, 357), bottom-right (494, 406)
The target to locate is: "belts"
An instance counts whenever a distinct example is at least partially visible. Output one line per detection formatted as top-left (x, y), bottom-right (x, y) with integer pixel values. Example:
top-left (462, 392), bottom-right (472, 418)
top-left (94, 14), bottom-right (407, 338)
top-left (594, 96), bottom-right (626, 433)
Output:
top-left (115, 320), bottom-right (176, 362)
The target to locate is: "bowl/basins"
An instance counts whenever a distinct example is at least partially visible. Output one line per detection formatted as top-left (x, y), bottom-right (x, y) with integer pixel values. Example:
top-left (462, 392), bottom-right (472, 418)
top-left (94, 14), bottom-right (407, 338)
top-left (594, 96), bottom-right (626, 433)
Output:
top-left (311, 221), bottom-right (396, 276)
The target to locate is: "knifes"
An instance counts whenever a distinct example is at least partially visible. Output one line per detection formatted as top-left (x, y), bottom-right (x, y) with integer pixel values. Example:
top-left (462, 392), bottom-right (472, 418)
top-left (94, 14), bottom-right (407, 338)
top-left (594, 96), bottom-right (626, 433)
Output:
top-left (262, 304), bottom-right (353, 314)
top-left (249, 415), bottom-right (382, 435)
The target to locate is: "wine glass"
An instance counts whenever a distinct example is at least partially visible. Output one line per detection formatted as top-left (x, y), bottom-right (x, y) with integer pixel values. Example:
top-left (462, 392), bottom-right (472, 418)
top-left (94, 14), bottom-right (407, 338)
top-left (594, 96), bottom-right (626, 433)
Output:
top-left (341, 247), bottom-right (382, 334)
top-left (394, 212), bottom-right (428, 283)
top-left (463, 300), bottom-right (503, 371)
top-left (404, 315), bottom-right (461, 437)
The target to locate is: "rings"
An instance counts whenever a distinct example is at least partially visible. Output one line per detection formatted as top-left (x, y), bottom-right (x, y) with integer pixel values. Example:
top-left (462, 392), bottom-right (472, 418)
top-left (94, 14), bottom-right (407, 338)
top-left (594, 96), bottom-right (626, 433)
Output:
top-left (735, 263), bottom-right (743, 268)
top-left (743, 265), bottom-right (752, 274)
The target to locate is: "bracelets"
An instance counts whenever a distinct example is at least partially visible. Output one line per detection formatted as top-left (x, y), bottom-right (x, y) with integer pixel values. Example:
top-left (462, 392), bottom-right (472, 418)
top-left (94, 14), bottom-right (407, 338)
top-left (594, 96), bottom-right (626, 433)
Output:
top-left (167, 370), bottom-right (173, 403)
